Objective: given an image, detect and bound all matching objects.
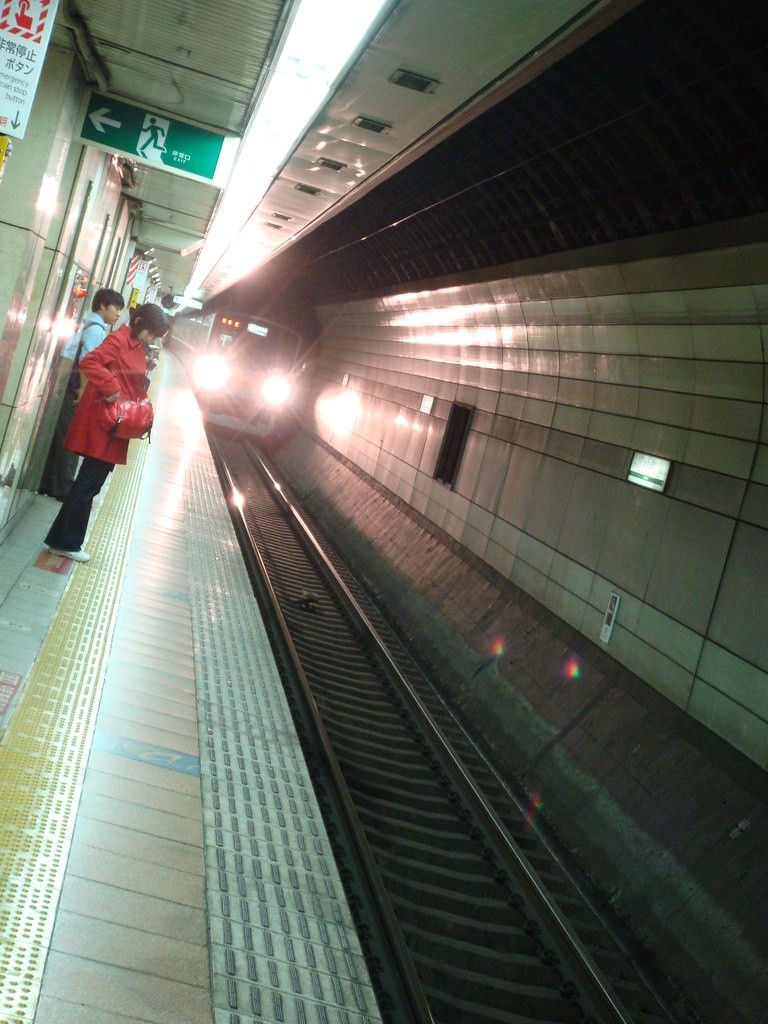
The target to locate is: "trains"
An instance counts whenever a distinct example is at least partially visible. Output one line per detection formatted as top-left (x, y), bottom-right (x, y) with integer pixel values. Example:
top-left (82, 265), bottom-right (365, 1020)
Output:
top-left (165, 306), bottom-right (304, 444)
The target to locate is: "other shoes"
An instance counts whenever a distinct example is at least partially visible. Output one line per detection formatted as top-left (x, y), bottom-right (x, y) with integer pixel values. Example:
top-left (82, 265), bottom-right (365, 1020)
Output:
top-left (38, 489), bottom-right (55, 497)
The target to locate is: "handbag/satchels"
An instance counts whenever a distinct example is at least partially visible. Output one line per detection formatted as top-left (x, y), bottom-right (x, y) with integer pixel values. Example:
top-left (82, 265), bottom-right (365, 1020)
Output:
top-left (98, 388), bottom-right (154, 444)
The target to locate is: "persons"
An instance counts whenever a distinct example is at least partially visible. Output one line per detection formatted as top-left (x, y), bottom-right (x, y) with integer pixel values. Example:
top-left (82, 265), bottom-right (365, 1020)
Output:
top-left (44, 304), bottom-right (169, 562)
top-left (37, 289), bottom-right (124, 503)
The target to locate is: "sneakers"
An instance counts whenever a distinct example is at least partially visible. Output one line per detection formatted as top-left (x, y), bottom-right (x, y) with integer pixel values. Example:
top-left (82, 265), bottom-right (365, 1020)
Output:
top-left (48, 546), bottom-right (90, 562)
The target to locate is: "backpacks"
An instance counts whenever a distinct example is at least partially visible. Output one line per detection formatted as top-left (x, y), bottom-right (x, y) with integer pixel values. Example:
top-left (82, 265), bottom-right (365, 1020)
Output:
top-left (68, 322), bottom-right (105, 389)
